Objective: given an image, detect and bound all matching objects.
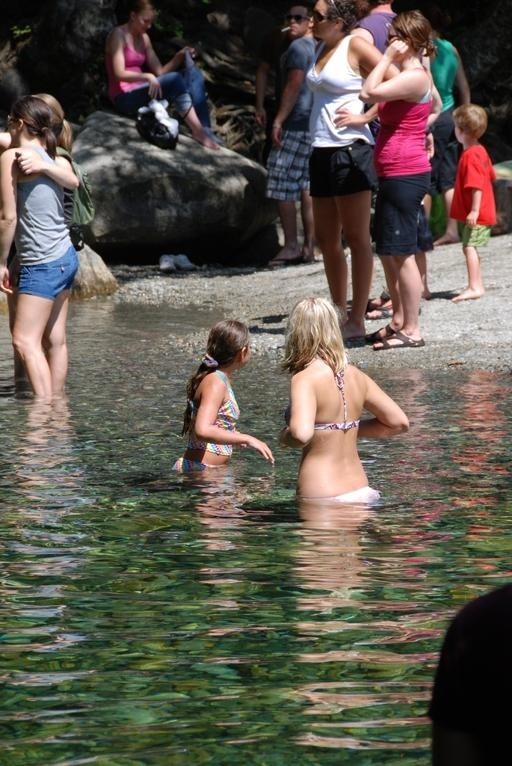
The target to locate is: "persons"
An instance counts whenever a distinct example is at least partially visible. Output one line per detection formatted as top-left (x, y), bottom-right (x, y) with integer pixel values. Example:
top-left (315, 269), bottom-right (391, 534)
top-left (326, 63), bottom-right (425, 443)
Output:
top-left (102, 0), bottom-right (226, 152)
top-left (261, 0), bottom-right (471, 267)
top-left (304, 1), bottom-right (443, 349)
top-left (277, 296), bottom-right (411, 506)
top-left (168, 318), bottom-right (278, 474)
top-left (449, 102), bottom-right (497, 303)
top-left (425, 578), bottom-right (512, 766)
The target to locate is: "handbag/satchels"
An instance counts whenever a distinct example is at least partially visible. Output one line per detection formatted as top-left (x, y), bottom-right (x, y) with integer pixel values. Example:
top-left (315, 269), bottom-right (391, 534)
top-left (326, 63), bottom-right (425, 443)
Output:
top-left (136, 112), bottom-right (178, 149)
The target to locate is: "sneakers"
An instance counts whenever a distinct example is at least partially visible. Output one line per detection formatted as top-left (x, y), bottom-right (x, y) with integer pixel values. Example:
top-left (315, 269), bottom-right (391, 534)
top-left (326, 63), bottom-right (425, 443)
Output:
top-left (175, 254), bottom-right (195, 271)
top-left (160, 254), bottom-right (176, 272)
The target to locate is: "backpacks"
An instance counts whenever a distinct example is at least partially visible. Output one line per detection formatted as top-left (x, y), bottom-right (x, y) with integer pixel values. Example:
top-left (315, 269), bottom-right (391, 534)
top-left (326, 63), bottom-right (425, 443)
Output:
top-left (55, 146), bottom-right (96, 226)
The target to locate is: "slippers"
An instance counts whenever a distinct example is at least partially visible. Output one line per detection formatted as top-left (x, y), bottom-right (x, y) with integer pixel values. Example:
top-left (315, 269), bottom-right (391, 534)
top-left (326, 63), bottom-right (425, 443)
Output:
top-left (265, 256), bottom-right (302, 266)
top-left (366, 288), bottom-right (425, 350)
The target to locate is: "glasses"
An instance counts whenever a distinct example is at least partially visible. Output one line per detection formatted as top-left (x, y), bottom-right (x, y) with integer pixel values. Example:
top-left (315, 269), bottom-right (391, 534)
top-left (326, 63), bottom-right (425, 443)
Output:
top-left (286, 15), bottom-right (310, 21)
